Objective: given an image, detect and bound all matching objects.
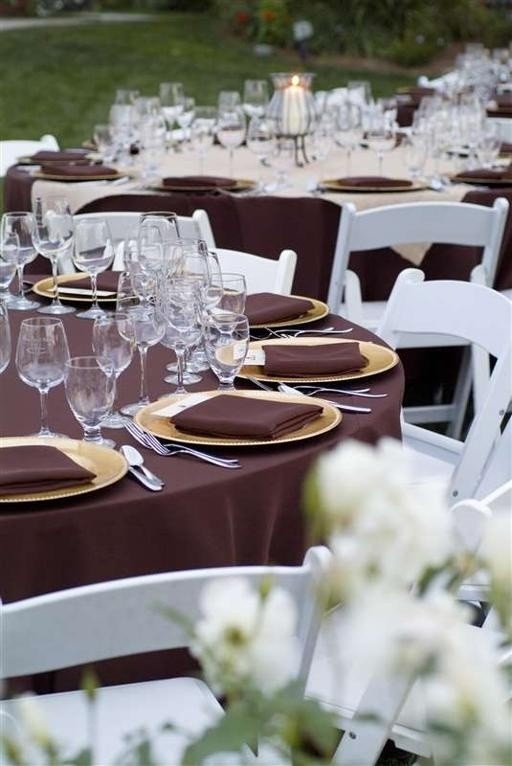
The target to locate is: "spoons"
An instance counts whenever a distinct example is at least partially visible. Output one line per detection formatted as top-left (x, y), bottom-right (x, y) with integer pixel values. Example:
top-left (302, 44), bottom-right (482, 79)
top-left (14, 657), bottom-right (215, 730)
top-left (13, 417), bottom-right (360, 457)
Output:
top-left (275, 382), bottom-right (372, 418)
top-left (121, 443), bottom-right (167, 490)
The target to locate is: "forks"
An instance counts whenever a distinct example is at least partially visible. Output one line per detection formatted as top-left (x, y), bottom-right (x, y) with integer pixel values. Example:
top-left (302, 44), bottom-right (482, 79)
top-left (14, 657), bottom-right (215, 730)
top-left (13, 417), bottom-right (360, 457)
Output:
top-left (232, 324), bottom-right (355, 339)
top-left (121, 421), bottom-right (242, 471)
top-left (242, 374), bottom-right (389, 403)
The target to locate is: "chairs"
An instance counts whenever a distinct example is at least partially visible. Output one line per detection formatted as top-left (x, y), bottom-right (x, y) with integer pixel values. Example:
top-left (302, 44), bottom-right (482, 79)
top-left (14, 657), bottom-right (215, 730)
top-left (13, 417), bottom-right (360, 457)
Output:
top-left (0, 74), bottom-right (511, 766)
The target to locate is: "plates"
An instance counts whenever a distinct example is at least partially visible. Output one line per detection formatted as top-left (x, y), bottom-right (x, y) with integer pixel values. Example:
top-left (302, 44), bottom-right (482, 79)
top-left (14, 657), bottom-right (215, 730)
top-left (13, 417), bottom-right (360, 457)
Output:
top-left (211, 338), bottom-right (401, 384)
top-left (134, 390), bottom-right (346, 455)
top-left (0, 434), bottom-right (126, 502)
top-left (196, 291), bottom-right (329, 332)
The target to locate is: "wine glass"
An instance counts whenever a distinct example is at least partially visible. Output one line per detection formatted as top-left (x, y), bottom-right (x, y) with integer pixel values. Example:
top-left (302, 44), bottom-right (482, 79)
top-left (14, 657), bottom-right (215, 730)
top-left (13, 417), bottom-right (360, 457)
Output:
top-left (1, 197), bottom-right (252, 448)
top-left (88, 42), bottom-right (510, 194)
